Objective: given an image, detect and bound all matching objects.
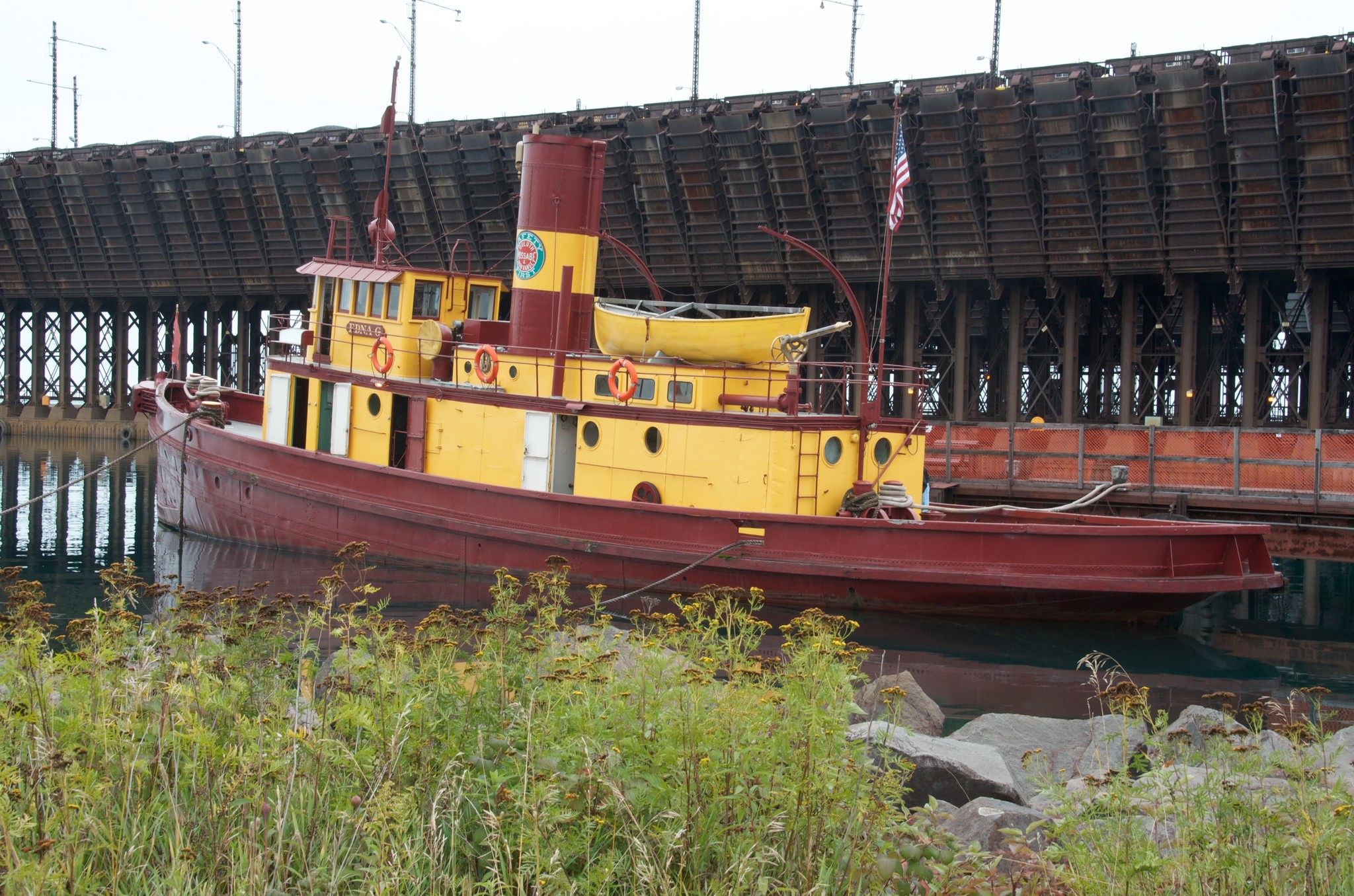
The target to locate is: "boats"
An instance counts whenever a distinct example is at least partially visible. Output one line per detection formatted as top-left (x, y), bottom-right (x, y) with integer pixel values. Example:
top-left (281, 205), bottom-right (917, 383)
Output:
top-left (132, 51), bottom-right (1284, 630)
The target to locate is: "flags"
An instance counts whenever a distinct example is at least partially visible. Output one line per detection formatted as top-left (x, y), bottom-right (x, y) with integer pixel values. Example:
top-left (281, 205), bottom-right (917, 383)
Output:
top-left (171, 311), bottom-right (181, 372)
top-left (886, 111), bottom-right (909, 232)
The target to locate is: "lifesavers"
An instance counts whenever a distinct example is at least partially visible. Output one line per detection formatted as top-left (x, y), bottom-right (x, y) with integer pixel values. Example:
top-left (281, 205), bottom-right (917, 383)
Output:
top-left (607, 358), bottom-right (639, 402)
top-left (370, 336), bottom-right (394, 375)
top-left (474, 343), bottom-right (499, 384)
